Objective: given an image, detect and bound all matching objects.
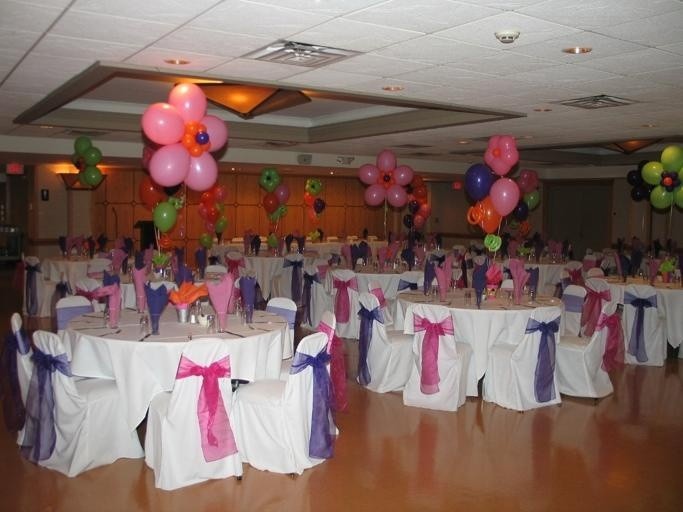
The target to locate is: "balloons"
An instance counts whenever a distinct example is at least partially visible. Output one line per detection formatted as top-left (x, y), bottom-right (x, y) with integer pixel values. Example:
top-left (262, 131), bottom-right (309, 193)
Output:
top-left (625, 144), bottom-right (682, 213)
top-left (138, 81), bottom-right (231, 264)
top-left (69, 136), bottom-right (107, 187)
top-left (358, 147), bottom-right (431, 238)
top-left (258, 162), bottom-right (291, 249)
top-left (462, 133), bottom-right (542, 253)
top-left (303, 175), bottom-right (326, 230)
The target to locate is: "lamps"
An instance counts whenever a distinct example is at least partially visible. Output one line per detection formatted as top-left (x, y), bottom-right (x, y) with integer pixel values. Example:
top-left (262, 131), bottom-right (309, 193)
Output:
top-left (61, 172), bottom-right (108, 190)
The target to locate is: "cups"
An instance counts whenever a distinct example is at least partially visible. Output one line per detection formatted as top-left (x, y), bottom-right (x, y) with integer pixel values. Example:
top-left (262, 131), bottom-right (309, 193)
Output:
top-left (106, 294), bottom-right (253, 334)
top-left (86, 261), bottom-right (202, 282)
top-left (423, 274), bottom-right (535, 308)
top-left (328, 256), bottom-right (406, 274)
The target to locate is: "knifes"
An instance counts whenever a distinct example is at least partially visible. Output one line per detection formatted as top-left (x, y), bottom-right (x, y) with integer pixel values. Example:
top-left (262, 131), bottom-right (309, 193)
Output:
top-left (225, 330), bottom-right (244, 337)
top-left (138, 333), bottom-right (150, 341)
